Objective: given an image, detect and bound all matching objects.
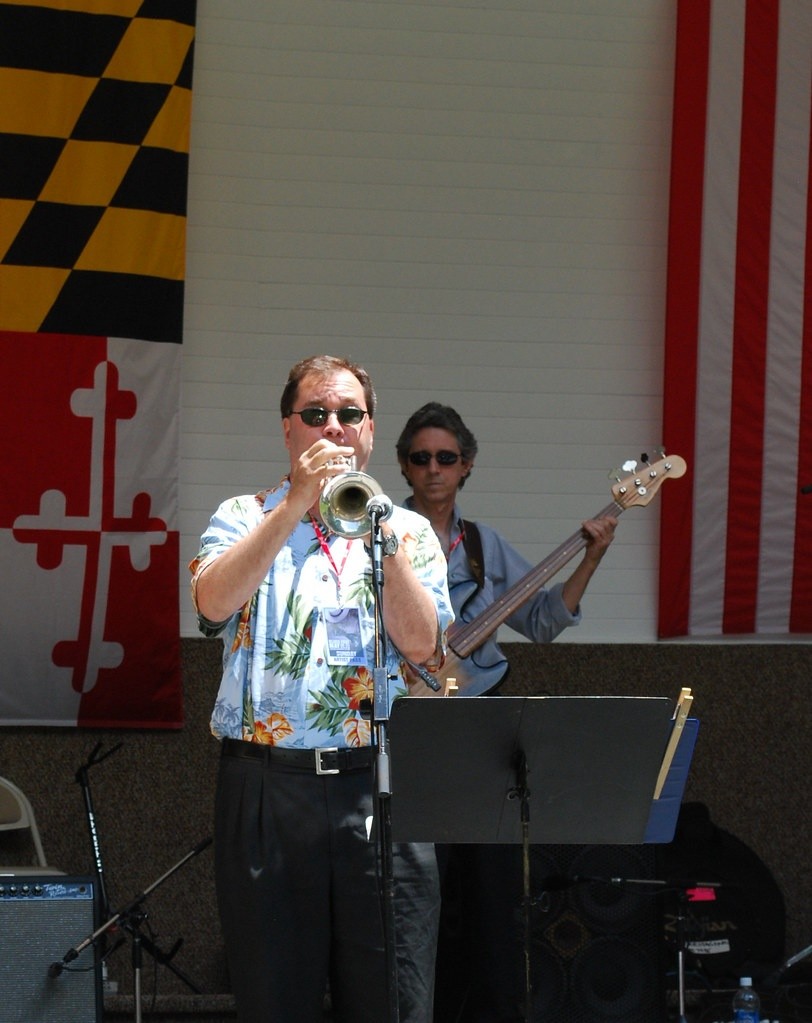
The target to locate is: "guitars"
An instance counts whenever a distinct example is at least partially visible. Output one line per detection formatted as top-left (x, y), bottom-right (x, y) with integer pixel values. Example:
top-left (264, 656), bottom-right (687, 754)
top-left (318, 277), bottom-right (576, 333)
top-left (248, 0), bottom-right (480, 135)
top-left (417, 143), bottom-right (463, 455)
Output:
top-left (401, 444), bottom-right (689, 697)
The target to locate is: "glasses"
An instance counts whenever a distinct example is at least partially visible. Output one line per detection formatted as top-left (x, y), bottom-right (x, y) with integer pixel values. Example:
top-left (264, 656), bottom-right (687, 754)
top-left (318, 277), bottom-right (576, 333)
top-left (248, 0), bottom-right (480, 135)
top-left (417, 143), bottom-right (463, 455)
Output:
top-left (291, 407), bottom-right (368, 426)
top-left (407, 449), bottom-right (462, 465)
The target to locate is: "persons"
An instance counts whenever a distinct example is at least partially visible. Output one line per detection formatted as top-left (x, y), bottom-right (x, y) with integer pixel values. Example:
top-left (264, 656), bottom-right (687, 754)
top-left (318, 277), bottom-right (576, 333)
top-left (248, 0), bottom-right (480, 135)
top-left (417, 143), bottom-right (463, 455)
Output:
top-left (376, 401), bottom-right (618, 1023)
top-left (189, 355), bottom-right (455, 1023)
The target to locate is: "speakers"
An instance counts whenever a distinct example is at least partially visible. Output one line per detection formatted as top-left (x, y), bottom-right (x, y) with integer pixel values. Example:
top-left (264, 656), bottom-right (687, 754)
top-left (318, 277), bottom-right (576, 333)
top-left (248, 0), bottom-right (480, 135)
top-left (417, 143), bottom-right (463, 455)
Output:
top-left (512, 838), bottom-right (671, 1023)
top-left (0, 875), bottom-right (103, 1023)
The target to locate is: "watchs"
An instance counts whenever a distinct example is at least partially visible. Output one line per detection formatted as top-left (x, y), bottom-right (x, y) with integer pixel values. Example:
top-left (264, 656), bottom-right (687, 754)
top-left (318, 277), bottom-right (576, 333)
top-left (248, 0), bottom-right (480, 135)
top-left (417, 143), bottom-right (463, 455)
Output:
top-left (364, 529), bottom-right (398, 558)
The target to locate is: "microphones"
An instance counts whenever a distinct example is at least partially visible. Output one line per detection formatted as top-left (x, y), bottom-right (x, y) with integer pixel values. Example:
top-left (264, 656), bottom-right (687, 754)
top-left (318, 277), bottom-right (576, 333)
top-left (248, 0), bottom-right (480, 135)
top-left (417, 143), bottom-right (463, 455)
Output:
top-left (47, 963), bottom-right (62, 978)
top-left (366, 494), bottom-right (393, 523)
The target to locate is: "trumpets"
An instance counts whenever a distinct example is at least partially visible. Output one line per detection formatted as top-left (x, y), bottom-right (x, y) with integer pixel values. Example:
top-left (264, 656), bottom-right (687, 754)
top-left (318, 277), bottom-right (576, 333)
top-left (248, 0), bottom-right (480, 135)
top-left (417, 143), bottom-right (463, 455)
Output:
top-left (319, 457), bottom-right (387, 541)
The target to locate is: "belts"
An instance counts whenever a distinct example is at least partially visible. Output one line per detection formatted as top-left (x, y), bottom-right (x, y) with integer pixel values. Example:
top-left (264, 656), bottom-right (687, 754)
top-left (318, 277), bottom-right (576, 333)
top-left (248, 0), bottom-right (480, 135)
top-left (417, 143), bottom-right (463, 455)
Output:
top-left (221, 739), bottom-right (378, 775)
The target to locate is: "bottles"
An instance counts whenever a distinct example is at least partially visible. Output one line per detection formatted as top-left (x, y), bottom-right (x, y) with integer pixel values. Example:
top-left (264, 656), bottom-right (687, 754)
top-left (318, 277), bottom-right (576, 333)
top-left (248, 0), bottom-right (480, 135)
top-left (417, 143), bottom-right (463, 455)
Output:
top-left (732, 977), bottom-right (760, 1023)
